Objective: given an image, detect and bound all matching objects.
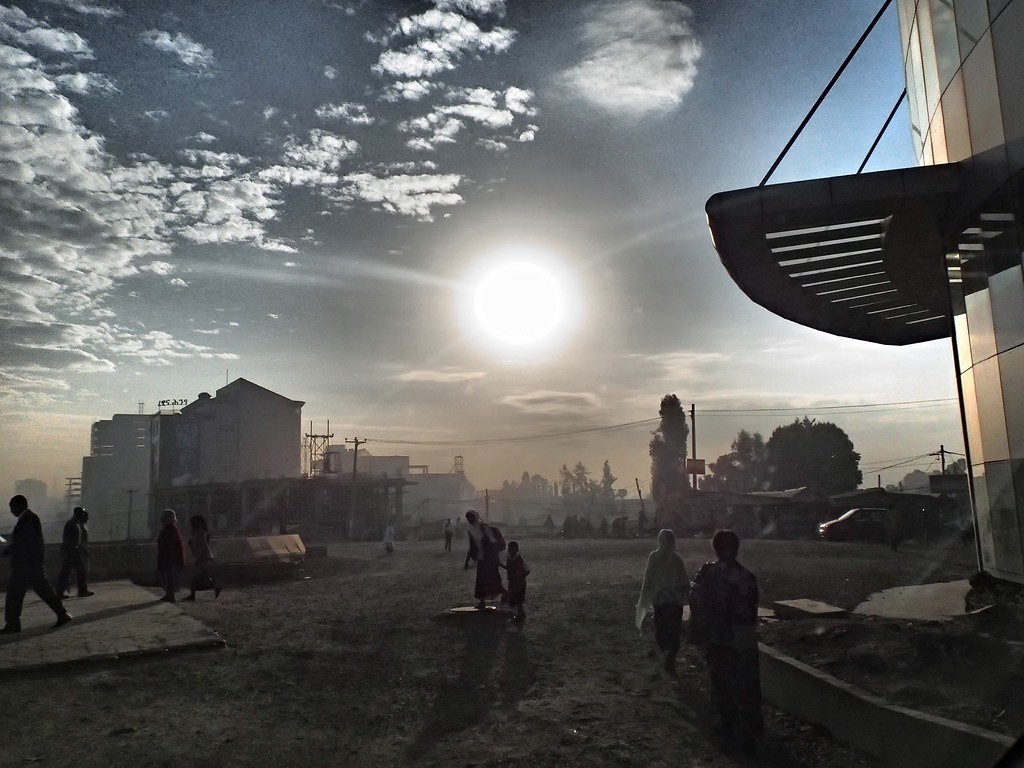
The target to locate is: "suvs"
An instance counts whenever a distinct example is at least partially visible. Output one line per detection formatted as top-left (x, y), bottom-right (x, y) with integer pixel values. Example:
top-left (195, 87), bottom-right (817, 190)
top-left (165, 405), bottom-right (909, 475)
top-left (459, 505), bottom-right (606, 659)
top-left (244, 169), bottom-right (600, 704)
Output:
top-left (818, 507), bottom-right (905, 541)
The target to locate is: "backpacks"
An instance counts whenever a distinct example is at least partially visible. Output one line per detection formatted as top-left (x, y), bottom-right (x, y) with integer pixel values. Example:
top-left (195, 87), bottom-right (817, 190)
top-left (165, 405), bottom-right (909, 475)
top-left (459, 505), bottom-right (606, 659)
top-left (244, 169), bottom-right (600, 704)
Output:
top-left (492, 526), bottom-right (506, 551)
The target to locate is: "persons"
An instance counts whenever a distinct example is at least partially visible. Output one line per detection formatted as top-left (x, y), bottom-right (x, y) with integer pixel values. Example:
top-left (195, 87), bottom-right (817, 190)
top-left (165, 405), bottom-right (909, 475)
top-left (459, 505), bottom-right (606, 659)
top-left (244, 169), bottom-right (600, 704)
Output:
top-left (454, 516), bottom-right (463, 540)
top-left (418, 516), bottom-right (424, 539)
top-left (155, 509), bottom-right (224, 603)
top-left (886, 502), bottom-right (901, 551)
top-left (507, 541), bottom-right (532, 625)
top-left (919, 506), bottom-right (936, 546)
top-left (279, 518), bottom-right (287, 533)
top-left (635, 528), bottom-right (692, 657)
top-left (542, 510), bottom-right (650, 537)
top-left (444, 518), bottom-right (453, 551)
top-left (462, 509), bottom-right (507, 609)
top-left (773, 505), bottom-right (787, 539)
top-left (0, 494), bottom-right (96, 633)
top-left (688, 530), bottom-right (764, 763)
top-left (385, 523), bottom-right (396, 553)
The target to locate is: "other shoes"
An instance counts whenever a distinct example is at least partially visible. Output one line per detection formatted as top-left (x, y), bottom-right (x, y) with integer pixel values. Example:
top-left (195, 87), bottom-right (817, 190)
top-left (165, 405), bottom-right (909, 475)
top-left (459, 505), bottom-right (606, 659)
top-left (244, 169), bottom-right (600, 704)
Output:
top-left (51, 611), bottom-right (72, 628)
top-left (501, 591), bottom-right (508, 602)
top-left (743, 738), bottom-right (758, 752)
top-left (512, 615), bottom-right (521, 624)
top-left (664, 660), bottom-right (677, 676)
top-left (521, 610), bottom-right (527, 617)
top-left (160, 596), bottom-right (175, 603)
top-left (214, 586), bottom-right (222, 599)
top-left (182, 595), bottom-right (195, 601)
top-left (0, 623), bottom-right (21, 633)
top-left (715, 719), bottom-right (733, 756)
top-left (79, 590), bottom-right (95, 597)
top-left (475, 601), bottom-right (485, 608)
top-left (56, 592), bottom-right (70, 599)
